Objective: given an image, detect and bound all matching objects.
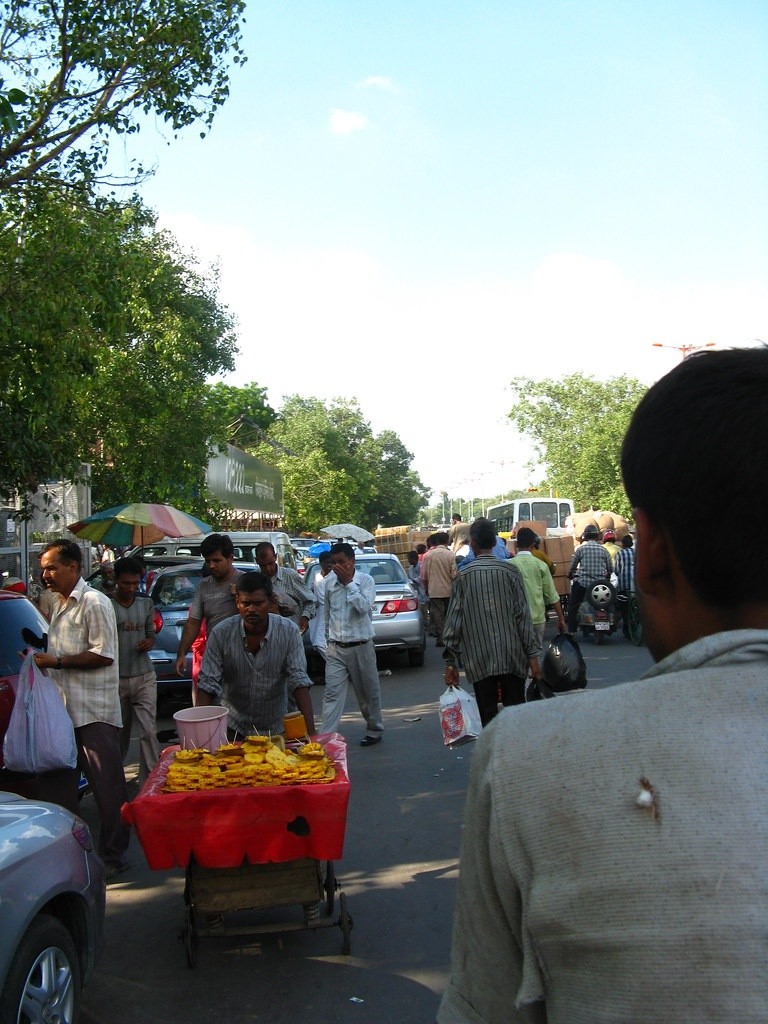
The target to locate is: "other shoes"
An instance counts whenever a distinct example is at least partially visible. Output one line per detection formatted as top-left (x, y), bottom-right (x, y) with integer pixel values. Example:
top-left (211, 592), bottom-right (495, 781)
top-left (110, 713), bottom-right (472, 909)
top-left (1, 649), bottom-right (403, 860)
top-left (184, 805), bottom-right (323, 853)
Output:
top-left (106, 854), bottom-right (129, 877)
top-left (361, 736), bottom-right (383, 746)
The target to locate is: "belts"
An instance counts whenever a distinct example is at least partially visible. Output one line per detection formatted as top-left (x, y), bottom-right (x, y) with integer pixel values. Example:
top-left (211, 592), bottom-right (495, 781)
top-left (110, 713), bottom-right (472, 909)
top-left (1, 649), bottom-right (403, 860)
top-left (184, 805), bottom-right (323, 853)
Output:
top-left (333, 641), bottom-right (367, 648)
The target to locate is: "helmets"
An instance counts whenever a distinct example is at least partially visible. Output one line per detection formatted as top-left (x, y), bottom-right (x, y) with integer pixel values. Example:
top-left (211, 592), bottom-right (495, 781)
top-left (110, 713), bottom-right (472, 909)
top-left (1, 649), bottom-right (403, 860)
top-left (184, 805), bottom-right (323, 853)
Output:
top-left (582, 524), bottom-right (599, 536)
top-left (602, 528), bottom-right (616, 542)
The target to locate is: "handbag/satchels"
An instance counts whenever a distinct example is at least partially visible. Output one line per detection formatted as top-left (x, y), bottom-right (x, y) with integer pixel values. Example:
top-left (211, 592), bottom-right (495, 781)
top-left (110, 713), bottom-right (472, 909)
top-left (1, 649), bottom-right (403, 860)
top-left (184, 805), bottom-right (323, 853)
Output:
top-left (439, 684), bottom-right (483, 748)
top-left (543, 631), bottom-right (587, 692)
top-left (3, 651), bottom-right (78, 771)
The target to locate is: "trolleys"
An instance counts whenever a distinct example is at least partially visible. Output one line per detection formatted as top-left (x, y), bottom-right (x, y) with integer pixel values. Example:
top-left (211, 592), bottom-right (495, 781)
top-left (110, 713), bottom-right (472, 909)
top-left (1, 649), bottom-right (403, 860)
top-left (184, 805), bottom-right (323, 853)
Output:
top-left (129, 731), bottom-right (354, 972)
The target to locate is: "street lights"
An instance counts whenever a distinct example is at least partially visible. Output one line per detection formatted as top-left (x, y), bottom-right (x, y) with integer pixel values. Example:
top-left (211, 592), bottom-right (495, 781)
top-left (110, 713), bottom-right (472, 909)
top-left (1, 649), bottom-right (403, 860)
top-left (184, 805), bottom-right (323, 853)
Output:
top-left (652, 342), bottom-right (716, 361)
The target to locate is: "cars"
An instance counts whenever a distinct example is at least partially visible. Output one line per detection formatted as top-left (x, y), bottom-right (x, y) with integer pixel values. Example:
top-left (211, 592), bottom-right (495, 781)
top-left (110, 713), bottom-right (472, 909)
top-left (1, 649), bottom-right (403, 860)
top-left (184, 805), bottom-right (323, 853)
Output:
top-left (145, 563), bottom-right (269, 717)
top-left (84, 555), bottom-right (206, 599)
top-left (302, 554), bottom-right (427, 670)
top-left (0, 589), bottom-right (92, 803)
top-left (0, 791), bottom-right (109, 1024)
top-left (282, 536), bottom-right (379, 582)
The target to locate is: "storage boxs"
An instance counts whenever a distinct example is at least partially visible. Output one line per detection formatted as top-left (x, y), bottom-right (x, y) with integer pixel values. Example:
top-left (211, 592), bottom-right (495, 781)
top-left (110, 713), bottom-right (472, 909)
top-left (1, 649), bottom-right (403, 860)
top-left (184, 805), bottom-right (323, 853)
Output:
top-left (508, 520), bottom-right (574, 595)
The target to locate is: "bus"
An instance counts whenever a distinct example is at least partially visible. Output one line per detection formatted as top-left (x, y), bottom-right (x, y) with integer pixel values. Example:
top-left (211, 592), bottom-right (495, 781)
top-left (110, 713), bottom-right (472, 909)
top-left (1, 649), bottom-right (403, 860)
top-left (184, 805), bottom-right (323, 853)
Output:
top-left (485, 498), bottom-right (575, 546)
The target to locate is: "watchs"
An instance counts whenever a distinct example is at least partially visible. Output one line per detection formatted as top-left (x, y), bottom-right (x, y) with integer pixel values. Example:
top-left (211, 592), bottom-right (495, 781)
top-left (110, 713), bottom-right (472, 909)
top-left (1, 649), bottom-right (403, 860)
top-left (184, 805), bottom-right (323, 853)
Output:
top-left (55, 655), bottom-right (62, 670)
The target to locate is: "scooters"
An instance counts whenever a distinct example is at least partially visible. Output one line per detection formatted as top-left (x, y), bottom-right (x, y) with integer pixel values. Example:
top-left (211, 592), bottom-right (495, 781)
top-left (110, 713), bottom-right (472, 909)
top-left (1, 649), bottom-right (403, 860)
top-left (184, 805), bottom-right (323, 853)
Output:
top-left (570, 571), bottom-right (618, 646)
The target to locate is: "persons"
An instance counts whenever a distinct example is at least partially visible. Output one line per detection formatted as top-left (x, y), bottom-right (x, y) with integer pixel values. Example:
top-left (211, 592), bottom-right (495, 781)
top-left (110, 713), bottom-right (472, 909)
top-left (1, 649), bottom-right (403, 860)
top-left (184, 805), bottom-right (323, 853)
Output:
top-left (318, 543), bottom-right (385, 747)
top-left (17, 538), bottom-right (131, 881)
top-left (174, 534), bottom-right (335, 713)
top-left (194, 572), bottom-right (317, 737)
top-left (438, 348), bottom-right (768, 1024)
top-left (85, 541), bottom-right (164, 604)
top-left (3, 576), bottom-right (25, 596)
top-left (106, 557), bottom-right (160, 786)
top-left (37, 571), bottom-right (65, 620)
top-left (408, 513), bottom-right (635, 665)
top-left (441, 518), bottom-right (543, 729)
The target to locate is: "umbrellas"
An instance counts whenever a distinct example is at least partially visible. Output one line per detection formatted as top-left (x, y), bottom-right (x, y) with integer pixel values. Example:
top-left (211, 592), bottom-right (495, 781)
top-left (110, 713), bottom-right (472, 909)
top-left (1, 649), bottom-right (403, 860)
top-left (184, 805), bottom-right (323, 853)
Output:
top-left (67, 501), bottom-right (214, 559)
top-left (319, 523), bottom-right (375, 543)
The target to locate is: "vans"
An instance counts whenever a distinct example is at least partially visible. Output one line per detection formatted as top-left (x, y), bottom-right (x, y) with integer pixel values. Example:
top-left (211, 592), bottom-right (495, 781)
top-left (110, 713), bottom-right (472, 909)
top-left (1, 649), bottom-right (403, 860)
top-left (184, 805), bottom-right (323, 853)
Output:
top-left (130, 531), bottom-right (299, 597)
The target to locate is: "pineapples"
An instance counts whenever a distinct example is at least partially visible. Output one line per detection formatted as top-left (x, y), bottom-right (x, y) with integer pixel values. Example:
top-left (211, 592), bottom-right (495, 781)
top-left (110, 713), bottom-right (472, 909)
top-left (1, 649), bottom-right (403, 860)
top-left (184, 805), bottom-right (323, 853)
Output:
top-left (166, 736), bottom-right (331, 794)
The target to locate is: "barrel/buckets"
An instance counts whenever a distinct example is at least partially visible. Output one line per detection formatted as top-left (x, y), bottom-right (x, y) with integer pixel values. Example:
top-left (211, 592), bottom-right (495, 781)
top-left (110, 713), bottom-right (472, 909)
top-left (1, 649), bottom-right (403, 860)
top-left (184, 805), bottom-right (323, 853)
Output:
top-left (174, 706), bottom-right (229, 752)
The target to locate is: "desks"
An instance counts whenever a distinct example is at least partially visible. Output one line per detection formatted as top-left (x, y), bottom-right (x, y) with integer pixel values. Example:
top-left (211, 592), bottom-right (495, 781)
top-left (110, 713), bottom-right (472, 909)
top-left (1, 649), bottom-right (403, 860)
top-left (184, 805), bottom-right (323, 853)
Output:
top-left (116, 731), bottom-right (350, 873)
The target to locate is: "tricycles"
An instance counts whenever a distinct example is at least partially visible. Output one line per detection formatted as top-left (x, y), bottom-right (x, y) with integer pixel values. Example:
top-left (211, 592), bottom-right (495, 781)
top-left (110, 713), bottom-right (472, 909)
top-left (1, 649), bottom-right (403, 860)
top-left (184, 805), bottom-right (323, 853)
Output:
top-left (624, 589), bottom-right (644, 647)
top-left (542, 594), bottom-right (571, 624)
